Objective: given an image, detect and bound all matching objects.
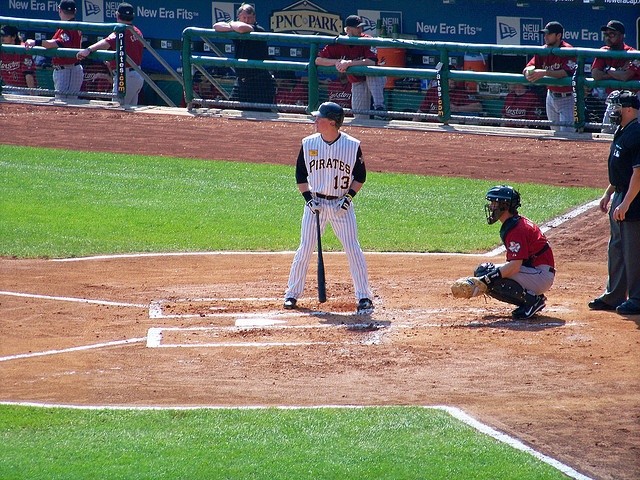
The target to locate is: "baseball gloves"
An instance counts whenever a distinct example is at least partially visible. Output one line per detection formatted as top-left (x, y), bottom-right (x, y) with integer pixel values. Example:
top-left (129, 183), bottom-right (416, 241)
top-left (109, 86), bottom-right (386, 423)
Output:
top-left (451, 276), bottom-right (491, 298)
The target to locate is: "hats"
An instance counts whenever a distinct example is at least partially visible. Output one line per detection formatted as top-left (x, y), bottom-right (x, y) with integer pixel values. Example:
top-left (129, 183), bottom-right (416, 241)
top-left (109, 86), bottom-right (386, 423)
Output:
top-left (117, 3), bottom-right (134, 16)
top-left (345, 15), bottom-right (366, 28)
top-left (0, 26), bottom-right (18, 36)
top-left (601, 20), bottom-right (625, 34)
top-left (538, 21), bottom-right (563, 34)
top-left (59, 0), bottom-right (76, 11)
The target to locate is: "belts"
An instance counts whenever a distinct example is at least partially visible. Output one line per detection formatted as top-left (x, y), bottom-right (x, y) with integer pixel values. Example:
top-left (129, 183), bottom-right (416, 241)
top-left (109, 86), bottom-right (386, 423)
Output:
top-left (549, 267), bottom-right (555, 274)
top-left (129, 68), bottom-right (135, 71)
top-left (549, 90), bottom-right (571, 97)
top-left (316, 192), bottom-right (338, 200)
top-left (53, 65), bottom-right (65, 70)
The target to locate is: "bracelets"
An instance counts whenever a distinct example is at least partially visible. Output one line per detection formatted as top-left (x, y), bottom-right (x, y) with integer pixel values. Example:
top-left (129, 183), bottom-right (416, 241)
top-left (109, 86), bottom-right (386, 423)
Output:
top-left (347, 189), bottom-right (356, 198)
top-left (87, 48), bottom-right (92, 53)
top-left (302, 191), bottom-right (312, 203)
top-left (605, 66), bottom-right (611, 74)
top-left (35, 40), bottom-right (42, 46)
top-left (487, 269), bottom-right (502, 284)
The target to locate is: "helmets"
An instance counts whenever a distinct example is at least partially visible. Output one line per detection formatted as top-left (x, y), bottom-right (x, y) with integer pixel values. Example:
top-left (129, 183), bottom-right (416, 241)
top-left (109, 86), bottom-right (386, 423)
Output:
top-left (605, 89), bottom-right (639, 130)
top-left (484, 185), bottom-right (521, 224)
top-left (310, 101), bottom-right (344, 123)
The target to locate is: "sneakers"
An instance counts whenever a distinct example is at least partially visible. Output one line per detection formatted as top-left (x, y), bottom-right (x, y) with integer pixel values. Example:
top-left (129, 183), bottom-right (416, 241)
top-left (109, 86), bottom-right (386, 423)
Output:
top-left (511, 295), bottom-right (546, 319)
top-left (357, 298), bottom-right (372, 311)
top-left (283, 298), bottom-right (295, 309)
top-left (588, 299), bottom-right (616, 310)
top-left (538, 294), bottom-right (547, 302)
top-left (616, 302), bottom-right (639, 315)
top-left (374, 107), bottom-right (391, 120)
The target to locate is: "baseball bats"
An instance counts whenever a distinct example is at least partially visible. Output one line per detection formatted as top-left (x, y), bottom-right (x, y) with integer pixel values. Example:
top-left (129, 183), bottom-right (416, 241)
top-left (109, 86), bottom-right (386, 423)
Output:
top-left (315, 202), bottom-right (326, 302)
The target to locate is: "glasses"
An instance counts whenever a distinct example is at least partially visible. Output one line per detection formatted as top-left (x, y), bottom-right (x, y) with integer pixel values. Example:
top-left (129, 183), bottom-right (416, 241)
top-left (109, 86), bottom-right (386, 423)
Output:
top-left (604, 32), bottom-right (616, 38)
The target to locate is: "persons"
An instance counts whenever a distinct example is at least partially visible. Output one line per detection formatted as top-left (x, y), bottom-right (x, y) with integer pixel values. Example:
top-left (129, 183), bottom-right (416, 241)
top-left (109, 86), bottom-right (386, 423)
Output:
top-left (78, 61), bottom-right (112, 102)
top-left (588, 89), bottom-right (640, 314)
top-left (76, 3), bottom-right (145, 105)
top-left (451, 185), bottom-right (556, 320)
top-left (283, 102), bottom-right (374, 309)
top-left (181, 70), bottom-right (225, 110)
top-left (213, 4), bottom-right (274, 112)
top-left (522, 21), bottom-right (577, 132)
top-left (500, 82), bottom-right (542, 129)
top-left (413, 66), bottom-right (482, 122)
top-left (25, 0), bottom-right (83, 100)
top-left (275, 74), bottom-right (307, 114)
top-left (328, 78), bottom-right (354, 118)
top-left (314, 15), bottom-right (387, 120)
top-left (591, 21), bottom-right (640, 133)
top-left (0, 26), bottom-right (38, 96)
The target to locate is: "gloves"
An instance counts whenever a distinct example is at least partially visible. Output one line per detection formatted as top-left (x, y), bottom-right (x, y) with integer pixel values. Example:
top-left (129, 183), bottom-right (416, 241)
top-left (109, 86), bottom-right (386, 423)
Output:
top-left (336, 193), bottom-right (353, 216)
top-left (306, 199), bottom-right (321, 215)
